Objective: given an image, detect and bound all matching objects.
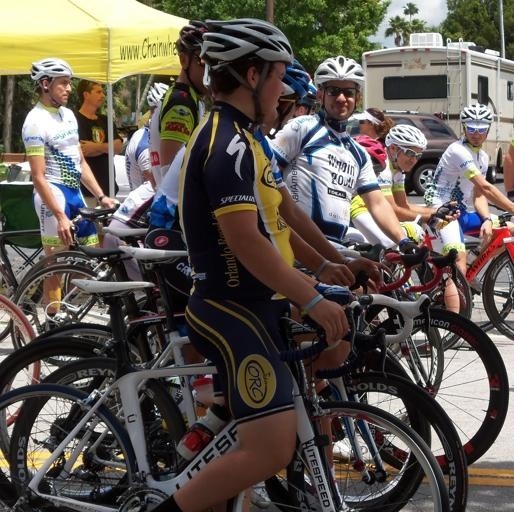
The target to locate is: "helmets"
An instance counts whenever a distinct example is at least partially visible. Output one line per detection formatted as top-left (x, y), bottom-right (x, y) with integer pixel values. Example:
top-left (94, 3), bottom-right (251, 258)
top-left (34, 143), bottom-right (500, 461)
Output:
top-left (174, 17), bottom-right (316, 106)
top-left (30, 58), bottom-right (72, 81)
top-left (460, 103), bottom-right (493, 129)
top-left (146, 82), bottom-right (169, 106)
top-left (385, 124), bottom-right (428, 150)
top-left (313, 55), bottom-right (365, 85)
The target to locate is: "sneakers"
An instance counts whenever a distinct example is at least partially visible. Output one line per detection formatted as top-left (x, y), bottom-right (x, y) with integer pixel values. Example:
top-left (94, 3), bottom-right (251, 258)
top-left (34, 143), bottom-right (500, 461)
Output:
top-left (175, 421), bottom-right (215, 461)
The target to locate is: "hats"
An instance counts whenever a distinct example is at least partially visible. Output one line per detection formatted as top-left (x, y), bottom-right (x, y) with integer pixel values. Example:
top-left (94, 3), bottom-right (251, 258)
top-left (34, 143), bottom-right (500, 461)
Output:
top-left (347, 110), bottom-right (381, 126)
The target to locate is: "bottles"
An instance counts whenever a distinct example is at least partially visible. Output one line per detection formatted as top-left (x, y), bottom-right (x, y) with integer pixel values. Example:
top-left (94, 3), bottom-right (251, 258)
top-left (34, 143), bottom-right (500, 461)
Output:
top-left (177, 401), bottom-right (231, 461)
top-left (193, 376), bottom-right (215, 417)
top-left (466, 248), bottom-right (479, 268)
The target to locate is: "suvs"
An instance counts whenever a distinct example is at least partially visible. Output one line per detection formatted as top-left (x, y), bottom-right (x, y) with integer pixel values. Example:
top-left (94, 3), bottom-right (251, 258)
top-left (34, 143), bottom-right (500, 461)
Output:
top-left (345, 109), bottom-right (460, 195)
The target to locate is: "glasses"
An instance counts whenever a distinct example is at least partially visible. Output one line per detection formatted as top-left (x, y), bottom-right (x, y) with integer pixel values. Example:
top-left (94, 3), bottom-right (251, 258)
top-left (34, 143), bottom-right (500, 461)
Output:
top-left (327, 86), bottom-right (359, 96)
top-left (464, 124), bottom-right (488, 134)
top-left (398, 145), bottom-right (423, 160)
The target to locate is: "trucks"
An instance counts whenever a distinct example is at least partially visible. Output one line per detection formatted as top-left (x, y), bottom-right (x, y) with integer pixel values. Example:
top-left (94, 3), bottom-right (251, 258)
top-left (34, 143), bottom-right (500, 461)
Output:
top-left (359, 29), bottom-right (514, 183)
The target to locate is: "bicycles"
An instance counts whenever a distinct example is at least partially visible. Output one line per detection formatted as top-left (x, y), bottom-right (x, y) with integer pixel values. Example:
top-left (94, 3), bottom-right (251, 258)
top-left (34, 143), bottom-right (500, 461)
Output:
top-left (1, 190), bottom-right (513, 512)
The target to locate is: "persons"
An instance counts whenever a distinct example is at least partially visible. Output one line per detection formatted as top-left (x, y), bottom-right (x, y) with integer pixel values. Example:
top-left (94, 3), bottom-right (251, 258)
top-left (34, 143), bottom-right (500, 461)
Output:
top-left (19, 19), bottom-right (514, 512)
